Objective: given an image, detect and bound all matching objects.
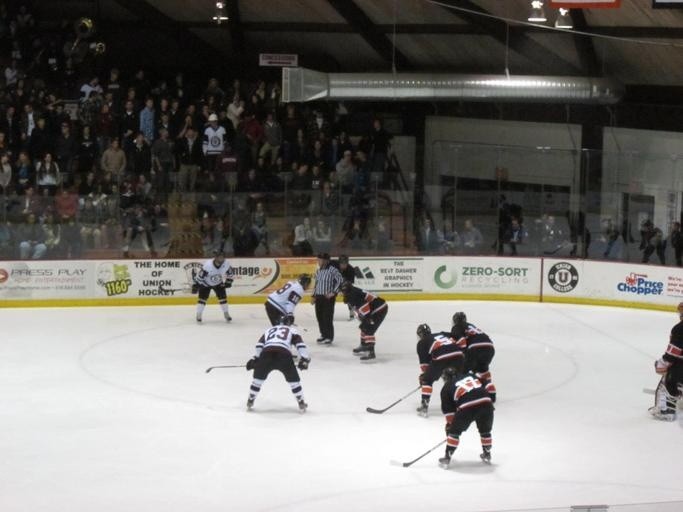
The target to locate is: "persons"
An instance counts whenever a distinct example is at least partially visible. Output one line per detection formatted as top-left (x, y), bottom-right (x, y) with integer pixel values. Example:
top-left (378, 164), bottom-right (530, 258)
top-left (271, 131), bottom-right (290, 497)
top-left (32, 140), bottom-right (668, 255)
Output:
top-left (416, 323), bottom-right (465, 414)
top-left (330, 253), bottom-right (356, 321)
top-left (655, 302), bottom-right (683, 422)
top-left (564, 209), bottom-right (683, 266)
top-left (440, 367), bottom-right (494, 464)
top-left (311, 252), bottom-right (343, 344)
top-left (341, 280), bottom-right (389, 360)
top-left (247, 315), bottom-right (311, 410)
top-left (264, 273), bottom-right (312, 327)
top-left (192, 249), bottom-right (234, 321)
top-left (451, 312), bottom-right (496, 372)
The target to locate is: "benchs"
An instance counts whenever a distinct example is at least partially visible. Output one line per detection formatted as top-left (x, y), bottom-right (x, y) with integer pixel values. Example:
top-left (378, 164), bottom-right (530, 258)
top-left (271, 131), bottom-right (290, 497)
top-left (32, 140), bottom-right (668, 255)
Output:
top-left (0, 167), bottom-right (411, 257)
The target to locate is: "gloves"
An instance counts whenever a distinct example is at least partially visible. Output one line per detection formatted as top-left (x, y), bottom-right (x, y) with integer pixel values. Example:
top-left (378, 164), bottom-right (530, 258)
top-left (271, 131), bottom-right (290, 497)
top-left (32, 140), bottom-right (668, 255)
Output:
top-left (298, 356), bottom-right (311, 369)
top-left (246, 356), bottom-right (258, 369)
top-left (225, 278), bottom-right (233, 287)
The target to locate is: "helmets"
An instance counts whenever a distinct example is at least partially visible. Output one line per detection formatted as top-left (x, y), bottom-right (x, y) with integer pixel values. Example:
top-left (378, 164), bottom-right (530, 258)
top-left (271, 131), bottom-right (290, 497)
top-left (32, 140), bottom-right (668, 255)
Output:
top-left (276, 315), bottom-right (291, 324)
top-left (340, 280), bottom-right (352, 292)
top-left (453, 311), bottom-right (466, 323)
top-left (300, 272), bottom-right (311, 290)
top-left (339, 254), bottom-right (348, 265)
top-left (417, 324), bottom-right (431, 337)
top-left (442, 366), bottom-right (459, 380)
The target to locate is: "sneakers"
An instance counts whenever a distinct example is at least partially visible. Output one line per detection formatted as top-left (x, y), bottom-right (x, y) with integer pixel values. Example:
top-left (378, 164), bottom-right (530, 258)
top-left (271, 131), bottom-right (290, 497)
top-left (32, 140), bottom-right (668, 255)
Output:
top-left (316, 336), bottom-right (331, 344)
top-left (225, 312), bottom-right (232, 321)
top-left (353, 346), bottom-right (375, 360)
top-left (247, 398), bottom-right (255, 407)
top-left (438, 456), bottom-right (451, 464)
top-left (299, 400), bottom-right (308, 409)
top-left (661, 408), bottom-right (676, 414)
top-left (196, 314), bottom-right (202, 322)
top-left (480, 452), bottom-right (490, 459)
top-left (416, 404), bottom-right (429, 412)
top-left (350, 310), bottom-right (357, 318)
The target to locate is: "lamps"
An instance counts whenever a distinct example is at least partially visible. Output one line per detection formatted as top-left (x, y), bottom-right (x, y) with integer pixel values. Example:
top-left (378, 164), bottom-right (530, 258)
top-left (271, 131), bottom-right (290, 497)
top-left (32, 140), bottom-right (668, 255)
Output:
top-left (209, 0), bottom-right (231, 26)
top-left (527, 0), bottom-right (548, 23)
top-left (554, 7), bottom-right (574, 29)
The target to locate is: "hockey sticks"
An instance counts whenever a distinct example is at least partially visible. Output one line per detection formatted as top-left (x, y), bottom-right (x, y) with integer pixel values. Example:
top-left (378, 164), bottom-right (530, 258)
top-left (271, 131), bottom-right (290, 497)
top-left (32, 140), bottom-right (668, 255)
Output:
top-left (207, 365), bottom-right (244, 372)
top-left (367, 388), bottom-right (422, 414)
top-left (389, 439), bottom-right (446, 467)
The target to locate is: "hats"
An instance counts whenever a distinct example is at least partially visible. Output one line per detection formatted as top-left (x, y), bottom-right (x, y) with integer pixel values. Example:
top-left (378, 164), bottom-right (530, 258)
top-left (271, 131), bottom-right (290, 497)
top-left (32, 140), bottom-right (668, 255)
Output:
top-left (317, 251), bottom-right (330, 259)
top-left (209, 114), bottom-right (218, 121)
